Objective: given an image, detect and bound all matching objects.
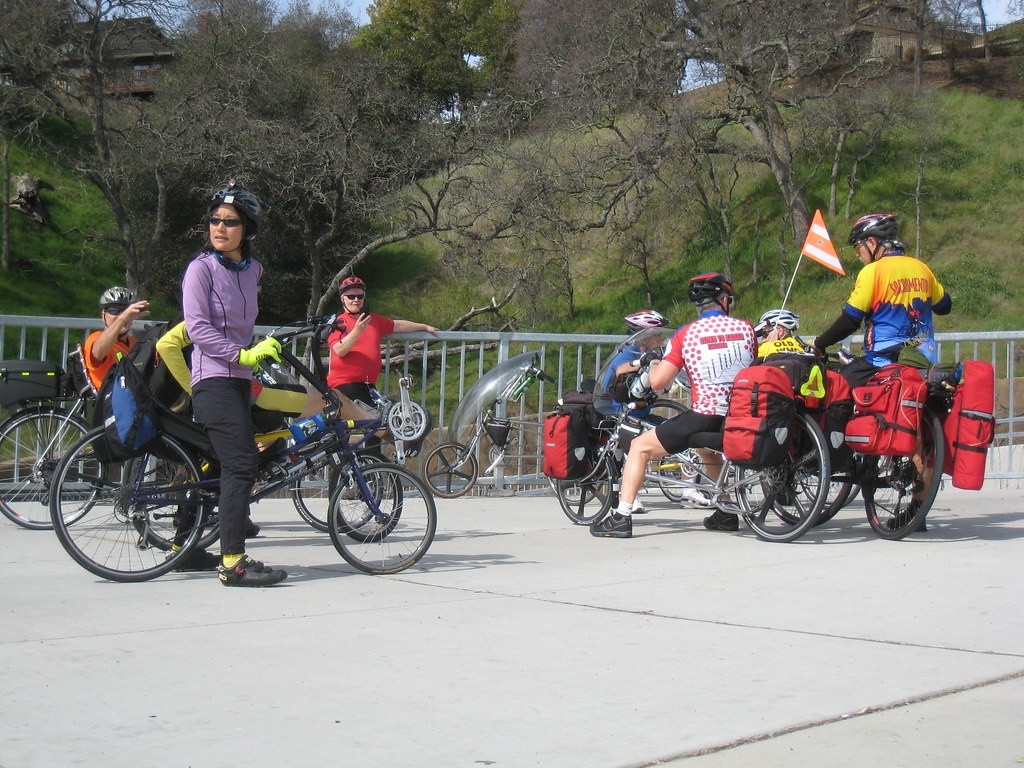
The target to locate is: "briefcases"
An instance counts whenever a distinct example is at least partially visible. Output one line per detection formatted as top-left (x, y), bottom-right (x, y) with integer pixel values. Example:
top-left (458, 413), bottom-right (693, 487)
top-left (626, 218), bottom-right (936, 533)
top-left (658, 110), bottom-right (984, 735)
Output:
top-left (0, 359), bottom-right (68, 409)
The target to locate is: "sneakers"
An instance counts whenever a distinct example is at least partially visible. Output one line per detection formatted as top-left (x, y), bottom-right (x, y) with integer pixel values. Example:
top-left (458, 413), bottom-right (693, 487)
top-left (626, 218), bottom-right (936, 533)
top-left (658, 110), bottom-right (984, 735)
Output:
top-left (680, 491), bottom-right (716, 509)
top-left (165, 512), bottom-right (288, 587)
top-left (886, 506), bottom-right (927, 533)
top-left (590, 509), bottom-right (633, 538)
top-left (703, 509), bottom-right (738, 531)
top-left (630, 500), bottom-right (644, 512)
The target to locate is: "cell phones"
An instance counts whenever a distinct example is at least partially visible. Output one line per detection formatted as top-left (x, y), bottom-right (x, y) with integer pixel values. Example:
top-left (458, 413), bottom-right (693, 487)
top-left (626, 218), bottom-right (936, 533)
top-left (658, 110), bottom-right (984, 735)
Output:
top-left (360, 306), bottom-right (370, 321)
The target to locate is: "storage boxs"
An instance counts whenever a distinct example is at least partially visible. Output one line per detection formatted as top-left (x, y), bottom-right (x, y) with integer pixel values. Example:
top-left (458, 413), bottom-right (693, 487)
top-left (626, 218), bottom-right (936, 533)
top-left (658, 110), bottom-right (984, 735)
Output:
top-left (0, 360), bottom-right (61, 407)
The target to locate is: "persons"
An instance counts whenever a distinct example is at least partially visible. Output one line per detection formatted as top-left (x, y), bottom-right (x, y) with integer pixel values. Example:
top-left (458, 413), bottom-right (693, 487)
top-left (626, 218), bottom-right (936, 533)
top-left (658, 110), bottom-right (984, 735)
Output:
top-left (753, 308), bottom-right (805, 506)
top-left (325, 275), bottom-right (439, 457)
top-left (600, 310), bottom-right (717, 516)
top-left (166, 185), bottom-right (288, 588)
top-left (812, 213), bottom-right (951, 531)
top-left (590, 271), bottom-right (758, 536)
top-left (83, 285), bottom-right (261, 538)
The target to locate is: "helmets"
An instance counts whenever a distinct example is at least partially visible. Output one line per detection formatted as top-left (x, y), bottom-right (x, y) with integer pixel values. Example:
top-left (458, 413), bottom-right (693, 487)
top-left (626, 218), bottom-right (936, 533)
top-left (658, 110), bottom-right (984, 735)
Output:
top-left (753, 310), bottom-right (800, 337)
top-left (687, 272), bottom-right (732, 302)
top-left (100, 286), bottom-right (137, 304)
top-left (624, 310), bottom-right (670, 331)
top-left (208, 186), bottom-right (262, 225)
top-left (846, 213), bottom-right (898, 246)
top-left (338, 276), bottom-right (366, 295)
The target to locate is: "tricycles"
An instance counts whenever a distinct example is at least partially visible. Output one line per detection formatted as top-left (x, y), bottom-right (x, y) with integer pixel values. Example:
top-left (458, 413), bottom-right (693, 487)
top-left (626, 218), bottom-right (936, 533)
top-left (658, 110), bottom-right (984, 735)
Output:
top-left (421, 349), bottom-right (702, 500)
top-left (48, 313), bottom-right (438, 582)
top-left (0, 338), bottom-right (438, 532)
top-left (555, 322), bottom-right (947, 544)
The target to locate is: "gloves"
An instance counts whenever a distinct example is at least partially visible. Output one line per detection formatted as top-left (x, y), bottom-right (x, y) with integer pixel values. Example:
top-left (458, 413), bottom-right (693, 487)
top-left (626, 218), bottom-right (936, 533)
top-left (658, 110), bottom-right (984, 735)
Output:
top-left (640, 353), bottom-right (662, 366)
top-left (238, 336), bottom-right (282, 366)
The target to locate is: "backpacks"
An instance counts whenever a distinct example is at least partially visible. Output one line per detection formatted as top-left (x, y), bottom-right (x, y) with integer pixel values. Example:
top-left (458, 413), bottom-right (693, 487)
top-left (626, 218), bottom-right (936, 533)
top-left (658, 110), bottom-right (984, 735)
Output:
top-left (724, 352), bottom-right (995, 491)
top-left (89, 319), bottom-right (192, 462)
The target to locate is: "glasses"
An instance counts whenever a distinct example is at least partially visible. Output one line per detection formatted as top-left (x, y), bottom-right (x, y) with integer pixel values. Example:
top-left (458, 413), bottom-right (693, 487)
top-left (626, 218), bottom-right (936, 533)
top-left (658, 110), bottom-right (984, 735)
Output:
top-left (721, 295), bottom-right (732, 304)
top-left (104, 306), bottom-right (123, 315)
top-left (852, 238), bottom-right (867, 252)
top-left (343, 293), bottom-right (364, 300)
top-left (761, 328), bottom-right (774, 338)
top-left (207, 217), bottom-right (248, 227)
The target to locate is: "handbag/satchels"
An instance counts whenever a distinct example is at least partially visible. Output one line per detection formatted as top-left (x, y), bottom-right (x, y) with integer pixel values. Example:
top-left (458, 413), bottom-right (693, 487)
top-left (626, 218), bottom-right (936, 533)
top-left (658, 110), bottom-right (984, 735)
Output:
top-left (542, 392), bottom-right (623, 480)
